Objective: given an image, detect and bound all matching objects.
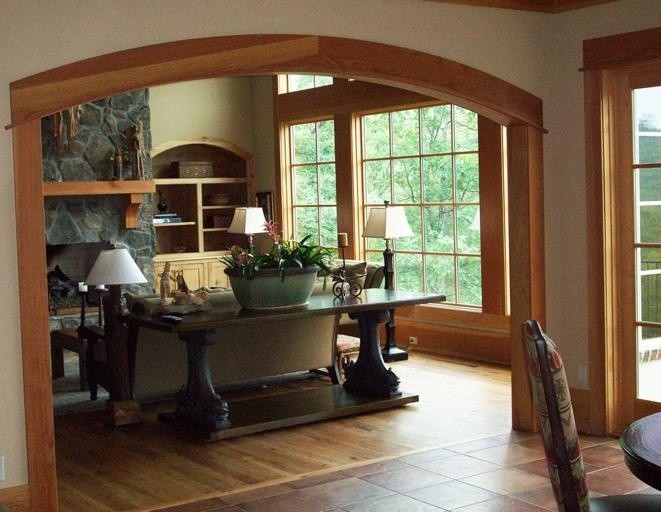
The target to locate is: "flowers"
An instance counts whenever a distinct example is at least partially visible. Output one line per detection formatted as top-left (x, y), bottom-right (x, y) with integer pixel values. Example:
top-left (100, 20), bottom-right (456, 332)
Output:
top-left (216, 220), bottom-right (339, 291)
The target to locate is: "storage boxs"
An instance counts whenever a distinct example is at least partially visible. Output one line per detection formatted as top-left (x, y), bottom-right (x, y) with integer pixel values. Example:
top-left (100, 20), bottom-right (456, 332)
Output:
top-left (171, 160), bottom-right (213, 178)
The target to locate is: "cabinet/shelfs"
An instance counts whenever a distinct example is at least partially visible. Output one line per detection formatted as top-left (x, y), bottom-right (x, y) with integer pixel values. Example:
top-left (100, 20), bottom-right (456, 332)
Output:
top-left (149, 135), bottom-right (255, 293)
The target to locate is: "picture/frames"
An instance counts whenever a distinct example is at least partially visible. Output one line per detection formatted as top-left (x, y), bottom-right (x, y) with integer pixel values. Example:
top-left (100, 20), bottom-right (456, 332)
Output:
top-left (254, 191), bottom-right (275, 225)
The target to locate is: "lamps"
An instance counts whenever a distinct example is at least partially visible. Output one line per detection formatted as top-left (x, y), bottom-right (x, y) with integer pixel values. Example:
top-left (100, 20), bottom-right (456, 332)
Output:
top-left (84, 248), bottom-right (148, 428)
top-left (227, 206), bottom-right (270, 255)
top-left (362, 206), bottom-right (415, 362)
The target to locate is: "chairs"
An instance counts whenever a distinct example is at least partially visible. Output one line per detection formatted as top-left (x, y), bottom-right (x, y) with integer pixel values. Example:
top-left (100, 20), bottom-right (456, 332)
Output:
top-left (521, 318), bottom-right (661, 512)
top-left (339, 263), bottom-right (385, 326)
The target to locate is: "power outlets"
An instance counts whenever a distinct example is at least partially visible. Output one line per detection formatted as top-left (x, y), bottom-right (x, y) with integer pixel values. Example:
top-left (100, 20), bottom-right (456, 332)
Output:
top-left (408, 336), bottom-right (418, 345)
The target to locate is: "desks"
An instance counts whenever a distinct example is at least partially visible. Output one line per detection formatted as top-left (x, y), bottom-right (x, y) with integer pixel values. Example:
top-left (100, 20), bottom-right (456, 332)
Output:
top-left (622, 408), bottom-right (661, 495)
top-left (120, 288), bottom-right (446, 443)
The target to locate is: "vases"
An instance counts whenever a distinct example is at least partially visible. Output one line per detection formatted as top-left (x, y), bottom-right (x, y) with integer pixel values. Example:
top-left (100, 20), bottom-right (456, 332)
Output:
top-left (224, 266), bottom-right (321, 311)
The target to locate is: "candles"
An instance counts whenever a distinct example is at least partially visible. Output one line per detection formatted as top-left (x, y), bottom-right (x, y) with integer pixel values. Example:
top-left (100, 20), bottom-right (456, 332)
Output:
top-left (78, 282), bottom-right (88, 292)
top-left (338, 232), bottom-right (348, 246)
top-left (96, 284), bottom-right (104, 290)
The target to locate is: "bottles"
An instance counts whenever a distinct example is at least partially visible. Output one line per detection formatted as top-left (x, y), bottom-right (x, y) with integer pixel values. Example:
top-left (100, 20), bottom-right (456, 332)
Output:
top-left (113, 143), bottom-right (123, 178)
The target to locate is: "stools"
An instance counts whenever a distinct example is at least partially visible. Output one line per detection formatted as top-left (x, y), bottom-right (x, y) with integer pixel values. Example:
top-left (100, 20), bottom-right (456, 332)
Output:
top-left (337, 334), bottom-right (361, 376)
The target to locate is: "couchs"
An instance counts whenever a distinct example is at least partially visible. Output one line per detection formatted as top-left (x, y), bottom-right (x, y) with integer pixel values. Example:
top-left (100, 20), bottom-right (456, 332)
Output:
top-left (78, 280), bottom-right (350, 405)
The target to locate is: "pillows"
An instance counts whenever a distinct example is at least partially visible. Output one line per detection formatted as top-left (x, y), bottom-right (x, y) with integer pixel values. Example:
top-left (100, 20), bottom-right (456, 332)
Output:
top-left (319, 260), bottom-right (367, 290)
